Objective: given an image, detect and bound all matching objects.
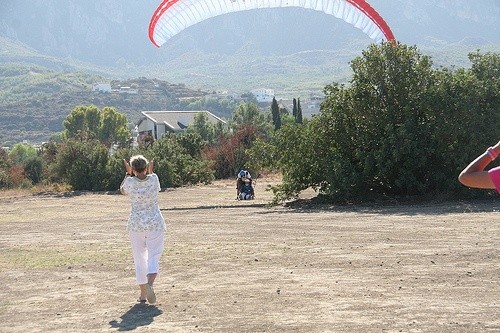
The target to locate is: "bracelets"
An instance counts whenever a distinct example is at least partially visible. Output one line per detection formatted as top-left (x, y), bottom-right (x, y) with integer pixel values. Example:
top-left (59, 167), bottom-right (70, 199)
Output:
top-left (487, 146), bottom-right (497, 160)
top-left (126, 171), bottom-right (132, 177)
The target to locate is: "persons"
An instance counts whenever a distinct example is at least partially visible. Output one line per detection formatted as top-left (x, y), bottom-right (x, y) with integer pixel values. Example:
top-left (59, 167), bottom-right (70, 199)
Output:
top-left (119, 154), bottom-right (168, 303)
top-left (458, 140), bottom-right (500, 194)
top-left (237, 170), bottom-right (252, 186)
top-left (237, 179), bottom-right (254, 199)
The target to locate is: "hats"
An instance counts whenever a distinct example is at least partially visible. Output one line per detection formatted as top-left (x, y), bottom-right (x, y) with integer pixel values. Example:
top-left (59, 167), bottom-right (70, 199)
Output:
top-left (240, 170), bottom-right (245, 176)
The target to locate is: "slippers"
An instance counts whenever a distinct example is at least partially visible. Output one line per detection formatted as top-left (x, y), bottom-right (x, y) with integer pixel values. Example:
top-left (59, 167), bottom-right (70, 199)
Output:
top-left (137, 297), bottom-right (147, 302)
top-left (144, 284), bottom-right (156, 303)
